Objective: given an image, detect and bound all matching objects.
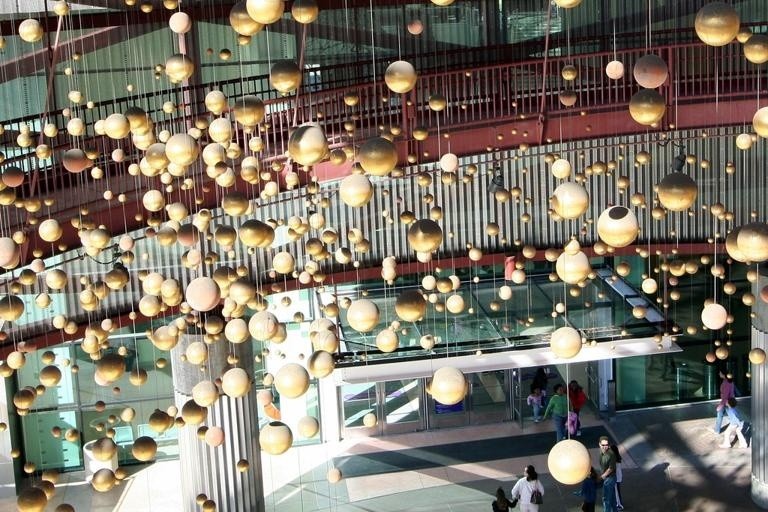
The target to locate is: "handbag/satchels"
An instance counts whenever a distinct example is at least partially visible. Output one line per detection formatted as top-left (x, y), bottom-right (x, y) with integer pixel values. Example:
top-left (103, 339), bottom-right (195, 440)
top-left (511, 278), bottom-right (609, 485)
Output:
top-left (530, 490), bottom-right (543, 504)
top-left (565, 411), bottom-right (577, 435)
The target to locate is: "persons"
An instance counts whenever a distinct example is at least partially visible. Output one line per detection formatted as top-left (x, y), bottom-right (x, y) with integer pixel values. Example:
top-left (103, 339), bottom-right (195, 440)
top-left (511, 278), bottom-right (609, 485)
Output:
top-left (717, 397), bottom-right (749, 449)
top-left (708, 370), bottom-right (735, 433)
top-left (492, 366), bottom-right (627, 511)
